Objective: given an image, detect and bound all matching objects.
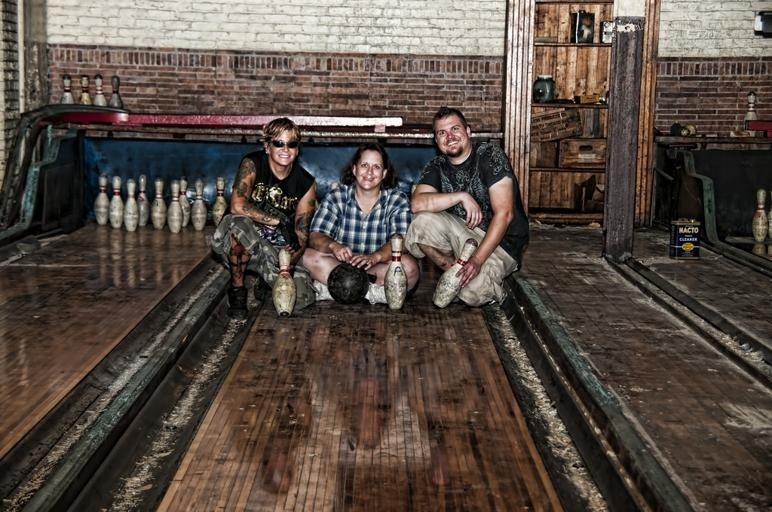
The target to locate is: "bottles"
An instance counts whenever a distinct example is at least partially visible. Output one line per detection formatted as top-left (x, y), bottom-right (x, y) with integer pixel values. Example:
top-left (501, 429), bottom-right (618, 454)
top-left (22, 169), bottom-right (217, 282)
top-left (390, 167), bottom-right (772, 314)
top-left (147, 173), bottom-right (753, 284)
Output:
top-left (533, 75), bottom-right (555, 104)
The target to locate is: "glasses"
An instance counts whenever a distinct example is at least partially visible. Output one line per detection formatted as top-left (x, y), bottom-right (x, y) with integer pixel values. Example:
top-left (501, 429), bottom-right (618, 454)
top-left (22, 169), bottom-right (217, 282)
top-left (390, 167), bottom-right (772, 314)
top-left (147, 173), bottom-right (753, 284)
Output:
top-left (269, 139), bottom-right (296, 148)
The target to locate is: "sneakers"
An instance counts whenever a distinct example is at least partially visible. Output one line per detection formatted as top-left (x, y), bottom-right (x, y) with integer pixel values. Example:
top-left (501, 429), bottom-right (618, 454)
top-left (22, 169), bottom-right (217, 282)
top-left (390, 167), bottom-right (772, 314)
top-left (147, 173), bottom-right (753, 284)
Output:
top-left (254, 274), bottom-right (267, 300)
top-left (366, 283), bottom-right (387, 304)
top-left (228, 283), bottom-right (248, 319)
top-left (313, 278), bottom-right (334, 301)
top-left (492, 289), bottom-right (508, 305)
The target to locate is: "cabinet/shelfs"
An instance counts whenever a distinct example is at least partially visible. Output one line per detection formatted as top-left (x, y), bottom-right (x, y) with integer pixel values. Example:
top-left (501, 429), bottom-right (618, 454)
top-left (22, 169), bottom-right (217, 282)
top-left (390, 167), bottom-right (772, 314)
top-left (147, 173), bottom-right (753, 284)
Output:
top-left (503, 0), bottom-right (662, 227)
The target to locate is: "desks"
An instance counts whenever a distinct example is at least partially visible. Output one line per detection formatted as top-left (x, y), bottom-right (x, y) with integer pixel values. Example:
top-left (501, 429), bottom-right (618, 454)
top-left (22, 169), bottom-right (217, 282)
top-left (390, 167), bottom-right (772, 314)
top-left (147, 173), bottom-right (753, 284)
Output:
top-left (655, 133), bottom-right (771, 226)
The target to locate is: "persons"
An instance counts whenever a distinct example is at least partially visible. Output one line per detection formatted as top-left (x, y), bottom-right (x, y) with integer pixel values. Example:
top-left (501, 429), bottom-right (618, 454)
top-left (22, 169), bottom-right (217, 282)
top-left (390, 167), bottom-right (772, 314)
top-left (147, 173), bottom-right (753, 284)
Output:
top-left (403, 106), bottom-right (530, 307)
top-left (295, 143), bottom-right (420, 306)
top-left (210, 117), bottom-right (320, 309)
